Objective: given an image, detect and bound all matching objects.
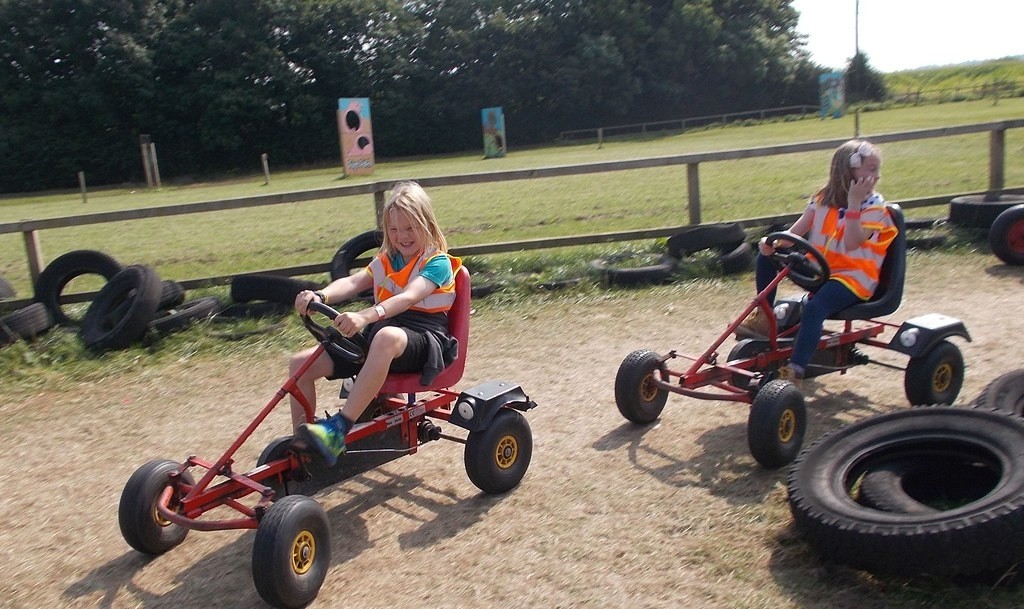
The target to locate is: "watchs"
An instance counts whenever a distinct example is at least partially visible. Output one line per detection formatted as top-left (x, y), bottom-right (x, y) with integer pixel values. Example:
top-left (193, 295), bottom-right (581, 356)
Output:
top-left (373, 304), bottom-right (386, 322)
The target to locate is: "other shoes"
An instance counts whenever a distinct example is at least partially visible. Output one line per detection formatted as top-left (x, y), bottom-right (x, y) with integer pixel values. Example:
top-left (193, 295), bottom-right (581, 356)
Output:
top-left (776, 366), bottom-right (804, 392)
top-left (728, 307), bottom-right (771, 340)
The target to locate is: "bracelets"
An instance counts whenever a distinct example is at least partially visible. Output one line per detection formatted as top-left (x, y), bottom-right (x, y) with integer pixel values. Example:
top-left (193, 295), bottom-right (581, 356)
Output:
top-left (775, 238), bottom-right (785, 250)
top-left (844, 210), bottom-right (862, 220)
top-left (313, 289), bottom-right (328, 306)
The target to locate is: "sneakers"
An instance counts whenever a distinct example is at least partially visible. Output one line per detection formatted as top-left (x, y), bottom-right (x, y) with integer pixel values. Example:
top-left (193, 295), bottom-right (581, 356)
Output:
top-left (298, 412), bottom-right (346, 467)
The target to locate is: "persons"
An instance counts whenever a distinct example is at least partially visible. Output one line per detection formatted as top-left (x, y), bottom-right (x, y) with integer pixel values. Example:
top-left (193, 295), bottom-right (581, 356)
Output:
top-left (725, 140), bottom-right (889, 391)
top-left (289, 179), bottom-right (458, 476)
